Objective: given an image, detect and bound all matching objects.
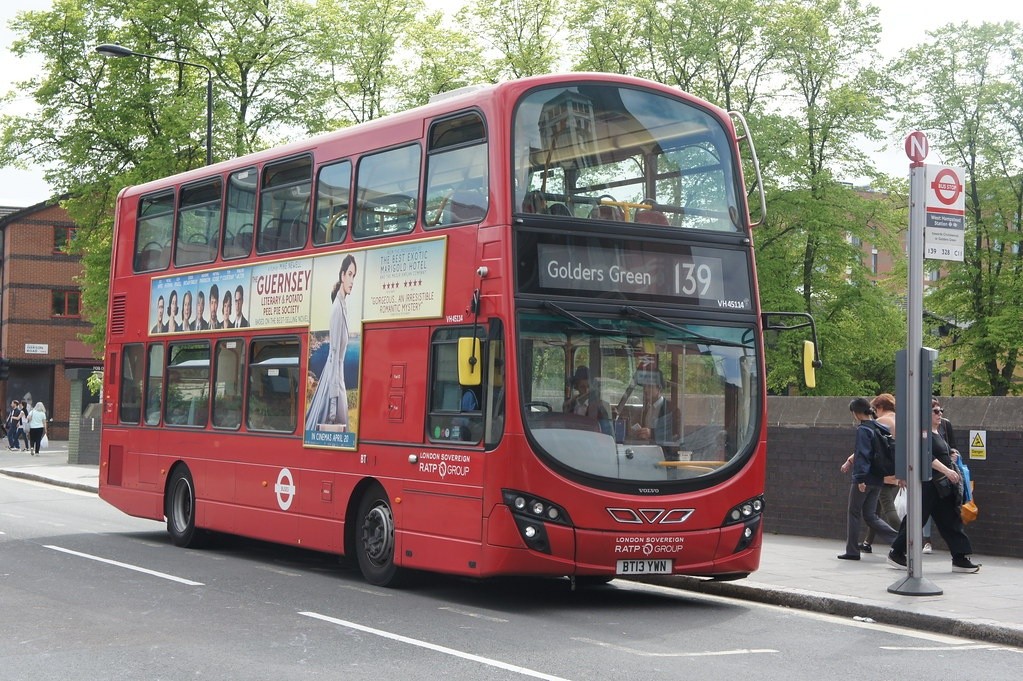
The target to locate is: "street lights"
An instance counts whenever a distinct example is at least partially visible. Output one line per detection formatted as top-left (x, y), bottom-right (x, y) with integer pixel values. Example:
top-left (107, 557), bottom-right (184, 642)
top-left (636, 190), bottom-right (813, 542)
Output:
top-left (94, 42), bottom-right (213, 166)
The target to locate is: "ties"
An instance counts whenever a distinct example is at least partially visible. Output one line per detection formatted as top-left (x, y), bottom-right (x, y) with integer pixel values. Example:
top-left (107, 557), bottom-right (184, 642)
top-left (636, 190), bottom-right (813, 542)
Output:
top-left (197, 324), bottom-right (199, 330)
top-left (235, 322), bottom-right (239, 327)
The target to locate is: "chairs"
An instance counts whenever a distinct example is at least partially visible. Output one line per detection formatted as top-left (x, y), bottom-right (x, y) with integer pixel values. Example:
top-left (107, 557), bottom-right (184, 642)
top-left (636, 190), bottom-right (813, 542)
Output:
top-left (136, 186), bottom-right (669, 272)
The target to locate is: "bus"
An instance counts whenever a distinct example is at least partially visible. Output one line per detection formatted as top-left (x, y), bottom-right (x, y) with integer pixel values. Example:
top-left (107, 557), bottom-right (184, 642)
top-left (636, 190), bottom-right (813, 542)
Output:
top-left (97, 72), bottom-right (824, 589)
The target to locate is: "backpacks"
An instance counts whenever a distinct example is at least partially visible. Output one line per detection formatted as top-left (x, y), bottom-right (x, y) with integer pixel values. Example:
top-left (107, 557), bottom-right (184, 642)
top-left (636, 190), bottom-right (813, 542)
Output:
top-left (861, 422), bottom-right (896, 477)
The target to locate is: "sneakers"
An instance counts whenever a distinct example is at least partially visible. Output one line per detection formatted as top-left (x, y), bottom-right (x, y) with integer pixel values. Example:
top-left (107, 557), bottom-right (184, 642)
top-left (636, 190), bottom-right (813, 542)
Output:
top-left (952, 556), bottom-right (979, 572)
top-left (888, 549), bottom-right (906, 569)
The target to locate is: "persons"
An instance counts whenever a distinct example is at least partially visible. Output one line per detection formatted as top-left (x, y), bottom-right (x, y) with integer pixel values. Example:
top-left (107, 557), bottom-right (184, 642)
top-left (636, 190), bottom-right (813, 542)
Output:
top-left (179, 291), bottom-right (193, 331)
top-left (563, 365), bottom-right (614, 436)
top-left (887, 399), bottom-right (979, 574)
top-left (837, 397), bottom-right (899, 561)
top-left (165, 290), bottom-right (179, 332)
top-left (233, 285), bottom-right (248, 328)
top-left (460, 357), bottom-right (504, 440)
top-left (633, 373), bottom-right (681, 461)
top-left (305, 255), bottom-right (356, 432)
top-left (220, 291), bottom-right (234, 328)
top-left (922, 396), bottom-right (957, 553)
top-left (27, 402), bottom-right (47, 454)
top-left (148, 394), bottom-right (188, 424)
top-left (4, 398), bottom-right (33, 452)
top-left (841, 393), bottom-right (901, 553)
top-left (191, 291), bottom-right (208, 330)
top-left (151, 295), bottom-right (167, 333)
top-left (207, 284), bottom-right (221, 329)
top-left (250, 405), bottom-right (274, 430)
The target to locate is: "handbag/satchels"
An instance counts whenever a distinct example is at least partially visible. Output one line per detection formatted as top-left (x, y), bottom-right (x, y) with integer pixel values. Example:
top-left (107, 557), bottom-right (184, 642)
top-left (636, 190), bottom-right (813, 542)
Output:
top-left (893, 486), bottom-right (908, 520)
top-left (23, 422), bottom-right (30, 434)
top-left (40, 432), bottom-right (49, 449)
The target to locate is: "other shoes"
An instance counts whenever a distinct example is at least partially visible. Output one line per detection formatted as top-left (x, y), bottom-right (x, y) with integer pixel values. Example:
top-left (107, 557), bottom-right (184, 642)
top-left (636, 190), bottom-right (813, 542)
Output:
top-left (31, 448), bottom-right (34, 455)
top-left (858, 542), bottom-right (873, 555)
top-left (23, 448), bottom-right (29, 451)
top-left (838, 553), bottom-right (861, 561)
top-left (923, 543), bottom-right (933, 553)
top-left (10, 447), bottom-right (21, 451)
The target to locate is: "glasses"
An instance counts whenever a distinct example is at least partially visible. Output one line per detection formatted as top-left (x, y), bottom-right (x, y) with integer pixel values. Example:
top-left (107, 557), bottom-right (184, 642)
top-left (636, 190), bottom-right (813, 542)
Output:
top-left (933, 409), bottom-right (944, 414)
